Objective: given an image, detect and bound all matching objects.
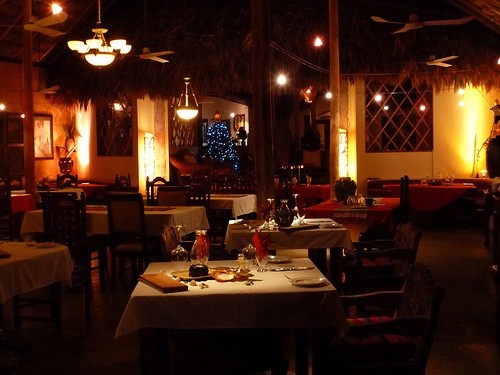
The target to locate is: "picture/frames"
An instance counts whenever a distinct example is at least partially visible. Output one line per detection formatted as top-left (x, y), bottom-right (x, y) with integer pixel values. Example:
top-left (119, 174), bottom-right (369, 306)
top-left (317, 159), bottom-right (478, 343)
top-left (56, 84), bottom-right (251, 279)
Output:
top-left (202, 119), bottom-right (208, 142)
top-left (33, 112), bottom-right (54, 159)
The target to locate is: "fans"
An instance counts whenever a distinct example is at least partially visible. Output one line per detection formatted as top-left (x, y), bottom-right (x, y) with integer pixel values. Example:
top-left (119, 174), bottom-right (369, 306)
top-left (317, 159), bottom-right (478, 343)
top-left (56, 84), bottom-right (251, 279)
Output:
top-left (398, 52), bottom-right (458, 67)
top-left (7, 36), bottom-right (60, 94)
top-left (371, 13), bottom-right (474, 33)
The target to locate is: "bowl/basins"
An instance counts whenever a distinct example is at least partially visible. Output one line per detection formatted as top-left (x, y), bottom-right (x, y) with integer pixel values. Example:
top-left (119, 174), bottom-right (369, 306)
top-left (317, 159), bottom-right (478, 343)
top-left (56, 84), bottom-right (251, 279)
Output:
top-left (189, 264), bottom-right (208, 276)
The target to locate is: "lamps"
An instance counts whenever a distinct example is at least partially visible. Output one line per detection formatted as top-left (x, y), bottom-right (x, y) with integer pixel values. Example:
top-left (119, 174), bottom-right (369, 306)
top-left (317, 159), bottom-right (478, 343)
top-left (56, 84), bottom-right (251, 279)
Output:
top-left (176, 76), bottom-right (199, 120)
top-left (68, 0), bottom-right (131, 67)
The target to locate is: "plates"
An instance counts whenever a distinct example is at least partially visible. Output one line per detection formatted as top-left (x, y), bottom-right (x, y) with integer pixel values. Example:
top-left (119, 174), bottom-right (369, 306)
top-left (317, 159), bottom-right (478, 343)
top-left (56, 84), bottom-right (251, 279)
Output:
top-left (291, 276), bottom-right (326, 286)
top-left (265, 255), bottom-right (292, 264)
top-left (172, 269), bottom-right (218, 280)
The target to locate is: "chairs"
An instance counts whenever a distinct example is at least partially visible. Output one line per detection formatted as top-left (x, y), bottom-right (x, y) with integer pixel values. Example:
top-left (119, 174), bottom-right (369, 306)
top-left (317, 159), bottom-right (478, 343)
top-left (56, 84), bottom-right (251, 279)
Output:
top-left (0, 172), bottom-right (499, 375)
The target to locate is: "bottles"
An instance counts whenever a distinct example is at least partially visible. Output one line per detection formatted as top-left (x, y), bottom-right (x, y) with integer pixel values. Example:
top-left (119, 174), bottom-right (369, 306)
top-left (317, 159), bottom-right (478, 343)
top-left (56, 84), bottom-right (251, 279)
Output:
top-left (334, 176), bottom-right (356, 203)
top-left (279, 199), bottom-right (291, 226)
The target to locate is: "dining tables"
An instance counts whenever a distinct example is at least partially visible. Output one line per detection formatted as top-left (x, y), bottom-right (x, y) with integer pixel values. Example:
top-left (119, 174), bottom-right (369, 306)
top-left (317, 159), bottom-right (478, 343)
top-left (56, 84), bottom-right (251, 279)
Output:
top-left (385, 182), bottom-right (476, 231)
top-left (0, 240), bottom-right (70, 346)
top-left (193, 193), bottom-right (257, 242)
top-left (10, 193), bottom-right (36, 240)
top-left (224, 215), bottom-right (355, 275)
top-left (109, 258), bottom-right (336, 375)
top-left (20, 205), bottom-right (210, 294)
top-left (51, 182), bottom-right (107, 205)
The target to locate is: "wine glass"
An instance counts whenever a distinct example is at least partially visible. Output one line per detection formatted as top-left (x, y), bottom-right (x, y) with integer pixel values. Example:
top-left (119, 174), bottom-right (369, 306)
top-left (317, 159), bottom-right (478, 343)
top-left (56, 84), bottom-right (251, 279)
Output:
top-left (171, 223), bottom-right (189, 271)
top-left (265, 193), bottom-right (301, 227)
top-left (191, 229), bottom-right (209, 266)
